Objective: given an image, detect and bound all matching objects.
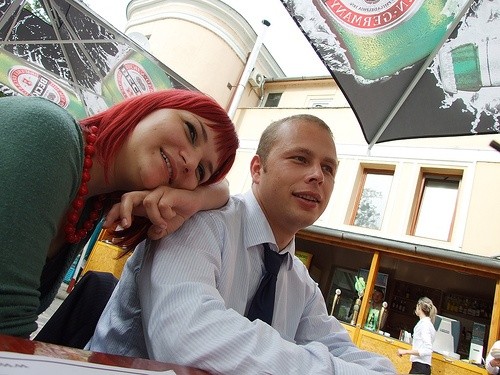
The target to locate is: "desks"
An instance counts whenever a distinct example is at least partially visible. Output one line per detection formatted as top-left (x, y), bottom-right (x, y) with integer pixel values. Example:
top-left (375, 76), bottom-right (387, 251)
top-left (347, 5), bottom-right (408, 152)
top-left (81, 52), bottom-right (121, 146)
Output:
top-left (0, 334), bottom-right (211, 375)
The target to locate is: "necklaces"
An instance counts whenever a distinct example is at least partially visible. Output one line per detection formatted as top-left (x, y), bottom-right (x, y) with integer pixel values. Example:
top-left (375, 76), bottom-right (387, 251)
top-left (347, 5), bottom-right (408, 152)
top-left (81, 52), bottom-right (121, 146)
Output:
top-left (66, 126), bottom-right (106, 244)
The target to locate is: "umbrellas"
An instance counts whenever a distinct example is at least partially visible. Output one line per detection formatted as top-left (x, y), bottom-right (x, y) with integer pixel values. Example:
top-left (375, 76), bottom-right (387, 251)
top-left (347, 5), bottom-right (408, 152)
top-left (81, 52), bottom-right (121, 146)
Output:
top-left (279, 0), bottom-right (500, 151)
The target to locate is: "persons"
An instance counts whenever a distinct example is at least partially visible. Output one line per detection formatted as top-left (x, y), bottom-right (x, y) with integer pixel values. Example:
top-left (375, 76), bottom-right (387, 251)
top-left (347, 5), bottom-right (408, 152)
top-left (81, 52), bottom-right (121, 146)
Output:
top-left (486, 341), bottom-right (500, 375)
top-left (373, 286), bottom-right (388, 330)
top-left (0, 91), bottom-right (239, 340)
top-left (397, 297), bottom-right (437, 375)
top-left (84, 114), bottom-right (396, 375)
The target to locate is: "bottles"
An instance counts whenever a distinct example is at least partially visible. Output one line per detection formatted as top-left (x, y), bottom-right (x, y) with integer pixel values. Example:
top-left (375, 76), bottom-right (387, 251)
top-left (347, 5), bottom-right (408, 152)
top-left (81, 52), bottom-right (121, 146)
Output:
top-left (0, 46), bottom-right (87, 121)
top-left (396, 282), bottom-right (402, 296)
top-left (406, 284), bottom-right (410, 298)
top-left (312, 0), bottom-right (464, 80)
top-left (445, 294), bottom-right (486, 318)
top-left (438, 37), bottom-right (500, 93)
top-left (101, 47), bottom-right (174, 108)
top-left (367, 313), bottom-right (375, 331)
top-left (392, 297), bottom-right (406, 312)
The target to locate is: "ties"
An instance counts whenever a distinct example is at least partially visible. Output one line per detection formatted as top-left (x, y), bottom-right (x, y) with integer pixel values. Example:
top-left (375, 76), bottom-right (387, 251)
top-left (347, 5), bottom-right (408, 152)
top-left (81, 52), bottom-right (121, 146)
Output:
top-left (247, 243), bottom-right (289, 327)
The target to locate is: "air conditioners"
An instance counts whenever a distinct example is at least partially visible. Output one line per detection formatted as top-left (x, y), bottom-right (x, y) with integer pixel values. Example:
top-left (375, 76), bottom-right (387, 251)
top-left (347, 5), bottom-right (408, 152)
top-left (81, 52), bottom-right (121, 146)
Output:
top-left (249, 68), bottom-right (264, 88)
